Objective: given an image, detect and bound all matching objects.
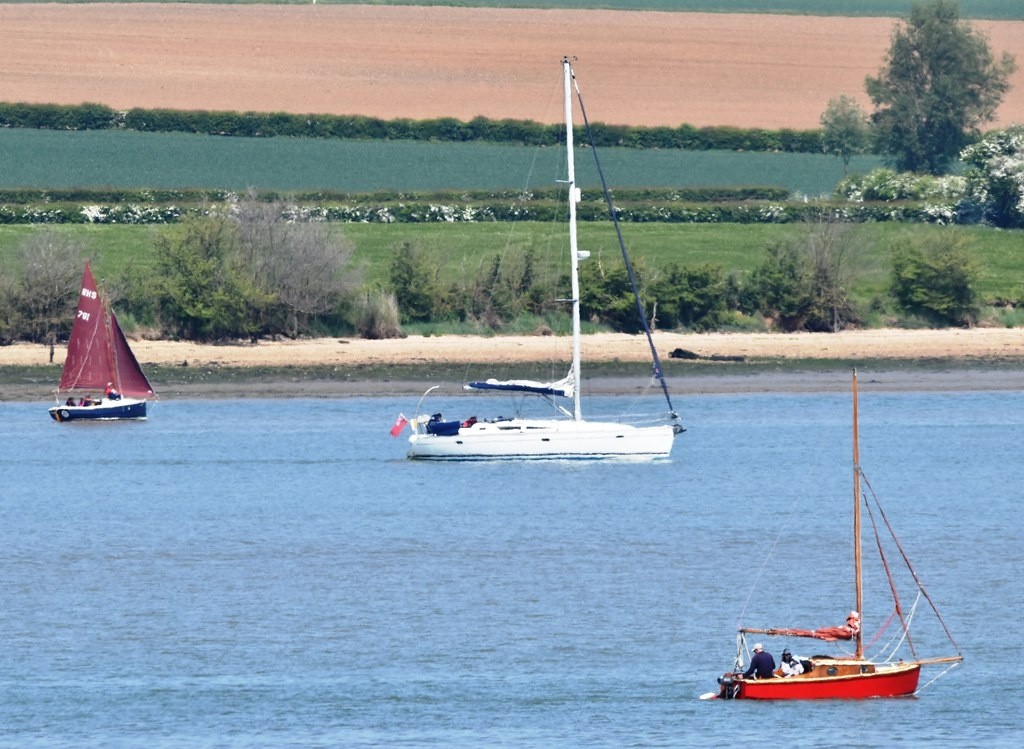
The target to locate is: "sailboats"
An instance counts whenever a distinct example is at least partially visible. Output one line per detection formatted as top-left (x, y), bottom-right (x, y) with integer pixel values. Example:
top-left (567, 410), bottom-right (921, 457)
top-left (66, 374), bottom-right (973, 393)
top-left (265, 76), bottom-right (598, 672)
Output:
top-left (700, 368), bottom-right (967, 705)
top-left (48, 258), bottom-right (156, 421)
top-left (406, 54), bottom-right (691, 463)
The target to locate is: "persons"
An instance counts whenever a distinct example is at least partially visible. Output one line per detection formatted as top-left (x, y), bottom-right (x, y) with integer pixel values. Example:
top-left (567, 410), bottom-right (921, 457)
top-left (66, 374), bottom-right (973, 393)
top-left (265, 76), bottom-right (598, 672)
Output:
top-left (737, 643), bottom-right (776, 679)
top-left (106, 382), bottom-right (121, 401)
top-left (79, 398), bottom-right (83, 406)
top-left (83, 395), bottom-right (93, 406)
top-left (780, 649), bottom-right (804, 678)
top-left (66, 397), bottom-right (76, 406)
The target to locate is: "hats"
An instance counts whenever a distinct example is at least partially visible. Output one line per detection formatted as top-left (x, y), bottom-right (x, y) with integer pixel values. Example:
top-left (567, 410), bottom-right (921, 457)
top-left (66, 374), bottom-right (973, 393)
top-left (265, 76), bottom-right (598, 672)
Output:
top-left (751, 643), bottom-right (762, 652)
top-left (782, 648), bottom-right (791, 655)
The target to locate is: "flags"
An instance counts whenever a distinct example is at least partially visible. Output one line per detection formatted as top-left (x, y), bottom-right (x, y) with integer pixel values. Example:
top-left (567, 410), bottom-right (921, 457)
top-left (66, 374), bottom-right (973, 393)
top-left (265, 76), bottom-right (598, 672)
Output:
top-left (390, 414), bottom-right (407, 437)
